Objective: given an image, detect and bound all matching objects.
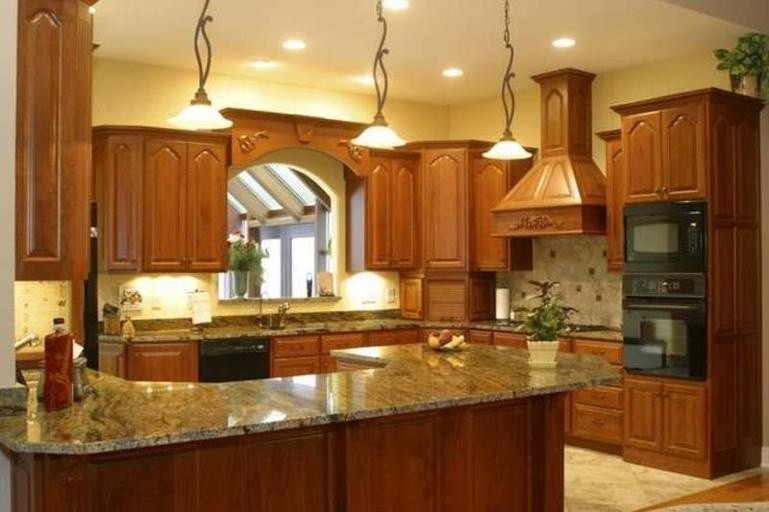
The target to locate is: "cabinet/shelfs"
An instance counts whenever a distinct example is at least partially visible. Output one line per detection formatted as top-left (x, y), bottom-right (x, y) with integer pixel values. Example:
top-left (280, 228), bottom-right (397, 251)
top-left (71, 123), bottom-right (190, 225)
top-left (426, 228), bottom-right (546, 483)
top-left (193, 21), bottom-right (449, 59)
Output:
top-left (552, 334), bottom-right (574, 436)
top-left (17, 2), bottom-right (94, 279)
top-left (593, 129), bottom-right (624, 273)
top-left (272, 322), bottom-right (365, 377)
top-left (607, 86), bottom-right (764, 481)
top-left (366, 323), bottom-right (421, 348)
top-left (99, 335), bottom-right (198, 383)
top-left (569, 337), bottom-right (623, 455)
top-left (468, 325), bottom-right (529, 350)
top-left (421, 323), bottom-right (467, 344)
top-left (345, 140), bottom-right (539, 322)
top-left (93, 124), bottom-right (230, 272)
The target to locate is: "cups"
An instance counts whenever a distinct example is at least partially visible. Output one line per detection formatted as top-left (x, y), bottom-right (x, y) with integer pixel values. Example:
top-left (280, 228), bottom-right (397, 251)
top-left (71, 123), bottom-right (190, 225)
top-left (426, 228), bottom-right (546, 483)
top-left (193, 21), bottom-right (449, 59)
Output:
top-left (317, 272), bottom-right (334, 297)
top-left (307, 277), bottom-right (313, 297)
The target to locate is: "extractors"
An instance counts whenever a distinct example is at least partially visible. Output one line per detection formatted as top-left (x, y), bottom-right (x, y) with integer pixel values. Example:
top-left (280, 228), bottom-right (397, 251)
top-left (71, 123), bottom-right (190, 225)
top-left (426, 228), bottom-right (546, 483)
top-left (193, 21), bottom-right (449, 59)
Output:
top-left (489, 67), bottom-right (609, 241)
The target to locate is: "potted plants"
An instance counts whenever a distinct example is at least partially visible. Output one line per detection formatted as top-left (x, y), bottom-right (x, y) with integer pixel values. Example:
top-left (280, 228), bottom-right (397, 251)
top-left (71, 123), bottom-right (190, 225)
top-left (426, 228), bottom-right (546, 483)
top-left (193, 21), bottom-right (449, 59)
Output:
top-left (227, 235), bottom-right (268, 296)
top-left (713, 31), bottom-right (769, 97)
top-left (510, 279), bottom-right (581, 369)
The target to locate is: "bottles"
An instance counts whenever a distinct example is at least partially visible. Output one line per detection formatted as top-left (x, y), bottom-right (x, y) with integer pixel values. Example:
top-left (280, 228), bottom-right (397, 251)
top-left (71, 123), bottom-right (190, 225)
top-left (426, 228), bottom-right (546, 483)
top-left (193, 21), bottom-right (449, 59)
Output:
top-left (71, 358), bottom-right (95, 403)
top-left (43, 317), bottom-right (74, 410)
top-left (120, 312), bottom-right (136, 335)
top-left (102, 301), bottom-right (122, 335)
top-left (36, 360), bottom-right (46, 401)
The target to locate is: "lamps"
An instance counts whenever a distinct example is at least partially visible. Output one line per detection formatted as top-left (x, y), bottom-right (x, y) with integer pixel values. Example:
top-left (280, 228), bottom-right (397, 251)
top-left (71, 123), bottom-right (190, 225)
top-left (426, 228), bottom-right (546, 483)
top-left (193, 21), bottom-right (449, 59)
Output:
top-left (483, 0), bottom-right (534, 160)
top-left (169, 0), bottom-right (233, 131)
top-left (352, 0), bottom-right (408, 145)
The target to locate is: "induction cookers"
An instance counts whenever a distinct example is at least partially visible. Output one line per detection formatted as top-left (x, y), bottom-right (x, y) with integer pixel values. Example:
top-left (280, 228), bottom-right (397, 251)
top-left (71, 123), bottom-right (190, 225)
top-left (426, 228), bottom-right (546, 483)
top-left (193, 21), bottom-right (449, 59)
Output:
top-left (497, 322), bottom-right (607, 334)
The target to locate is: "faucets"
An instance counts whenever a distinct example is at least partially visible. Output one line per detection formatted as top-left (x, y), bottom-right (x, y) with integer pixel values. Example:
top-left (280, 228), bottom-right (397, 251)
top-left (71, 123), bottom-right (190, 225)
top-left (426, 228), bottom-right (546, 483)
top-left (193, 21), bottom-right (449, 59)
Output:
top-left (15, 335), bottom-right (42, 352)
top-left (270, 302), bottom-right (289, 329)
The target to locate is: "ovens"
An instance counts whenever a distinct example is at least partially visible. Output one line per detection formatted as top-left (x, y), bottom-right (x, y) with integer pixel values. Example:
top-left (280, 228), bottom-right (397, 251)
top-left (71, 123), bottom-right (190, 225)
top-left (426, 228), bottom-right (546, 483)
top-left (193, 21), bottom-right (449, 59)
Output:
top-left (621, 200), bottom-right (710, 382)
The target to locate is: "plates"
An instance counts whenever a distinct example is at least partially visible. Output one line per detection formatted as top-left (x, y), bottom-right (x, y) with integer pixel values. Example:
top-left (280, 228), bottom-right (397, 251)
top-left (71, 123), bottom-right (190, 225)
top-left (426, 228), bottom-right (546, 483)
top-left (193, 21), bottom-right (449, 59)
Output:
top-left (421, 342), bottom-right (472, 353)
